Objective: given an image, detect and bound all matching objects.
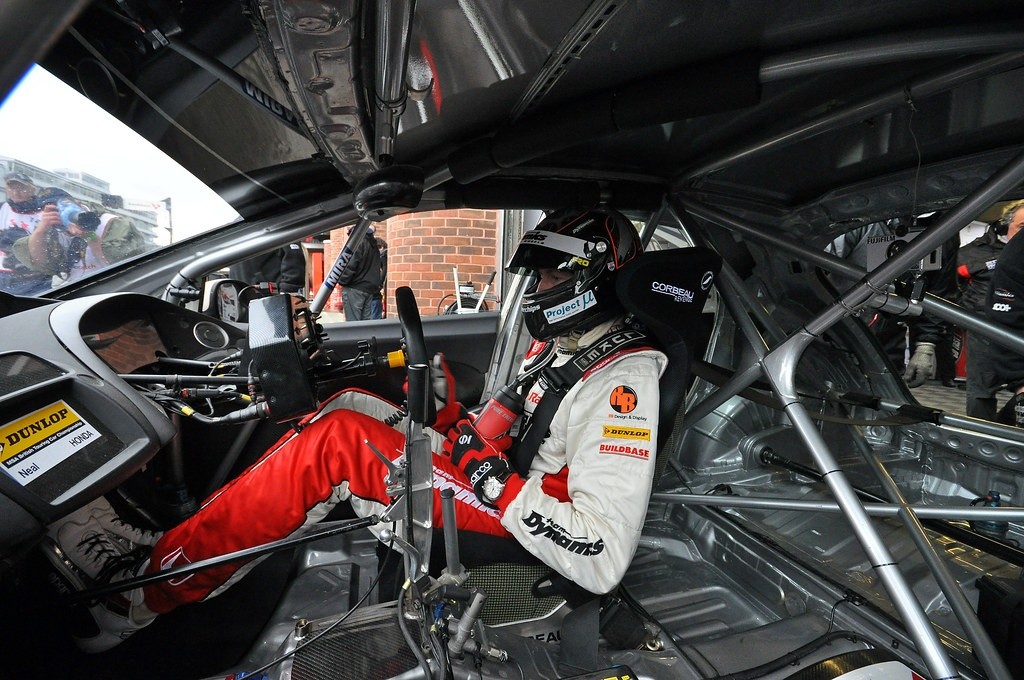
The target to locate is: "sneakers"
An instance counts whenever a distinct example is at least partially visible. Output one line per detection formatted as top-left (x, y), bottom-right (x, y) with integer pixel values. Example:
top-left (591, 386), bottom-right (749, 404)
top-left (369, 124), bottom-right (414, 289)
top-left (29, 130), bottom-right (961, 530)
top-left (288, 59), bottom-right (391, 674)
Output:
top-left (38, 505), bottom-right (159, 653)
top-left (87, 496), bottom-right (164, 577)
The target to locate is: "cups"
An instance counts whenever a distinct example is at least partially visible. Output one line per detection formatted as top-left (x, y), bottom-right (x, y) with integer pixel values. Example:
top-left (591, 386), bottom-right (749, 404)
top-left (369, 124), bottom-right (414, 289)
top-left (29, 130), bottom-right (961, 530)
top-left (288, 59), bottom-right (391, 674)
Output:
top-left (472, 379), bottom-right (526, 440)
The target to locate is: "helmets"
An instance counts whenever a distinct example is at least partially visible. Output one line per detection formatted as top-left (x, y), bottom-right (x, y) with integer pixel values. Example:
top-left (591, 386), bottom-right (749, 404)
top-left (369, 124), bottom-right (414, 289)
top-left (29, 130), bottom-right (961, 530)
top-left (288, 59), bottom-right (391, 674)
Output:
top-left (505, 204), bottom-right (645, 342)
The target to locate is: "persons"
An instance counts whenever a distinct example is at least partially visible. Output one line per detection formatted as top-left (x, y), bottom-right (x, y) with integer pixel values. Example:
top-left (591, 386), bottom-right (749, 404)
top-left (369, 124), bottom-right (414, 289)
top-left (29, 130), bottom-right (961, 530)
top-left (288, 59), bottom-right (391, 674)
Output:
top-left (0, 172), bottom-right (145, 296)
top-left (229, 243), bottom-right (306, 297)
top-left (342, 226), bottom-right (388, 321)
top-left (846, 199), bottom-right (1024, 429)
top-left (40, 207), bottom-right (669, 656)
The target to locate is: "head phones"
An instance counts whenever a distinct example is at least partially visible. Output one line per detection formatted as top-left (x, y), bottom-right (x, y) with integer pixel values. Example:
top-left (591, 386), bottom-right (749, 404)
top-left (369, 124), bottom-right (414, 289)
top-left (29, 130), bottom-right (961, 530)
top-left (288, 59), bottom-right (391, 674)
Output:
top-left (993, 203), bottom-right (1022, 235)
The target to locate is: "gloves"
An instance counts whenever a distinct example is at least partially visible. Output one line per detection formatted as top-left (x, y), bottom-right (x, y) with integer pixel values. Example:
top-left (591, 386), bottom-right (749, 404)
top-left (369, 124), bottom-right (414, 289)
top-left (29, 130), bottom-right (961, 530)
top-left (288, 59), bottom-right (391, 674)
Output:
top-left (402, 352), bottom-right (468, 437)
top-left (902, 341), bottom-right (937, 387)
top-left (441, 419), bottom-right (514, 510)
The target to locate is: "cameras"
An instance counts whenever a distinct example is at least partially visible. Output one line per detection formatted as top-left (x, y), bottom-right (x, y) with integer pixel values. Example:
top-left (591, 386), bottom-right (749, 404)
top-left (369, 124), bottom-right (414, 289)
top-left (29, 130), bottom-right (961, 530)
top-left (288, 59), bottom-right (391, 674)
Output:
top-left (54, 206), bottom-right (101, 232)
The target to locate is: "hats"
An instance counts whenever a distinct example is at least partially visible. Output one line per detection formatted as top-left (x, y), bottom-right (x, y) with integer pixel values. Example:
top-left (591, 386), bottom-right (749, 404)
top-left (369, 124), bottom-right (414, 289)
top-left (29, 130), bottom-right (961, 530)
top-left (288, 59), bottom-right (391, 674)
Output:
top-left (4, 172), bottom-right (36, 188)
top-left (37, 187), bottom-right (70, 209)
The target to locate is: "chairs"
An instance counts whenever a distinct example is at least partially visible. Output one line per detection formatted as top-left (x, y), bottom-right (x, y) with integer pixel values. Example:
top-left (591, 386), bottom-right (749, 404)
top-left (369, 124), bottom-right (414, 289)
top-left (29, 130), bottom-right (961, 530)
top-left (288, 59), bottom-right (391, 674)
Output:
top-left (406, 247), bottom-right (718, 626)
